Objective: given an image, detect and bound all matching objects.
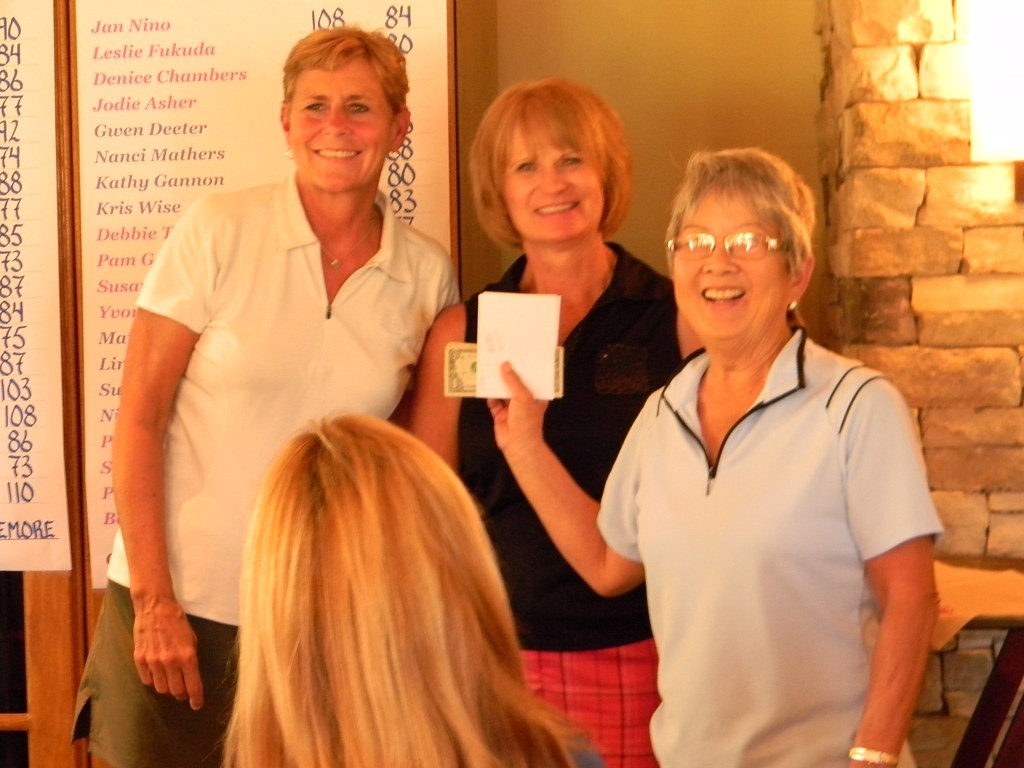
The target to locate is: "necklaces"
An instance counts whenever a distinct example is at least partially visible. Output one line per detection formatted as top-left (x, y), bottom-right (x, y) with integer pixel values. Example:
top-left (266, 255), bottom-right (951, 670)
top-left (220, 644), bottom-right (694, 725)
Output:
top-left (322, 207), bottom-right (375, 268)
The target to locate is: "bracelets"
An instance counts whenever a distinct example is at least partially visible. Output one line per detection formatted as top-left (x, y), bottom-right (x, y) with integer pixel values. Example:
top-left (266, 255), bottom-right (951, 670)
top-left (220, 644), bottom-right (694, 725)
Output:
top-left (848, 747), bottom-right (899, 765)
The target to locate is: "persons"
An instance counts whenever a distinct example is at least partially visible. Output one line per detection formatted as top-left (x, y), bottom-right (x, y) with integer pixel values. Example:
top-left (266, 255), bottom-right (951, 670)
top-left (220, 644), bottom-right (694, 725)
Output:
top-left (413, 75), bottom-right (703, 768)
top-left (488, 145), bottom-right (944, 768)
top-left (70, 29), bottom-right (458, 767)
top-left (223, 413), bottom-right (603, 768)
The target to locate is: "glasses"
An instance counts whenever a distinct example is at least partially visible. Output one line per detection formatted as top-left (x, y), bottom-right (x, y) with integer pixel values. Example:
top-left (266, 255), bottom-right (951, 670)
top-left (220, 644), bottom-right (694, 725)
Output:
top-left (668, 226), bottom-right (791, 261)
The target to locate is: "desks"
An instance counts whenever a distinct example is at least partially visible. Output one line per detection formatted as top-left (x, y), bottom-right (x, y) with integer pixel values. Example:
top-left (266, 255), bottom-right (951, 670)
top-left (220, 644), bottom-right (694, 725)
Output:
top-left (932, 557), bottom-right (1024, 630)
